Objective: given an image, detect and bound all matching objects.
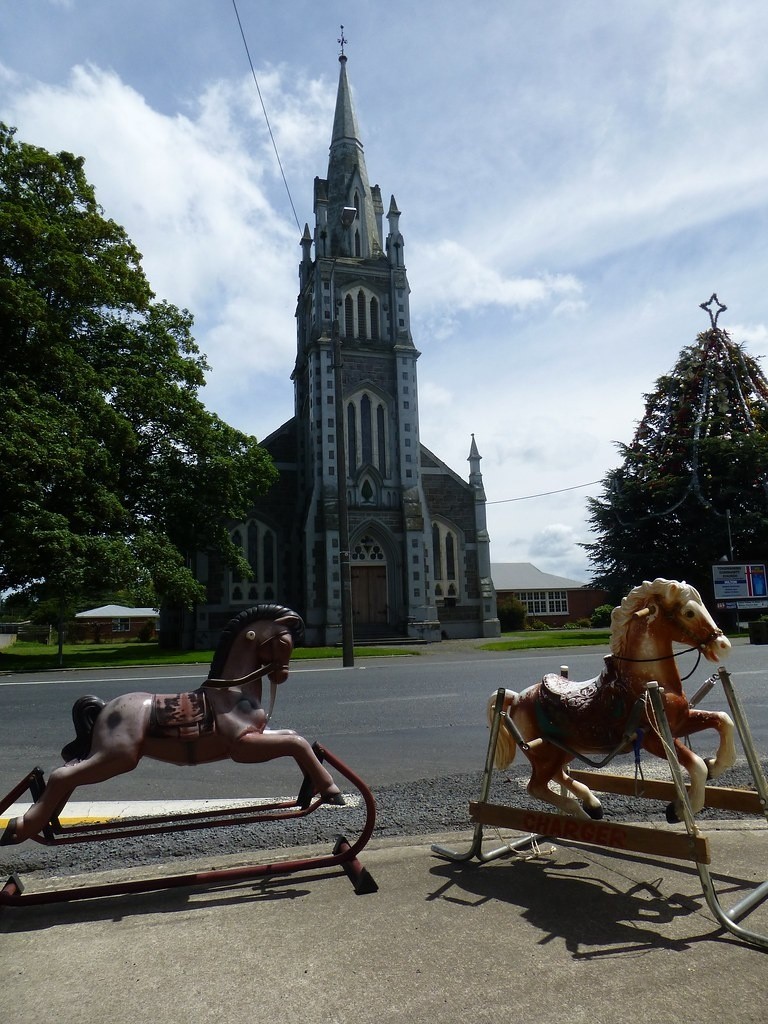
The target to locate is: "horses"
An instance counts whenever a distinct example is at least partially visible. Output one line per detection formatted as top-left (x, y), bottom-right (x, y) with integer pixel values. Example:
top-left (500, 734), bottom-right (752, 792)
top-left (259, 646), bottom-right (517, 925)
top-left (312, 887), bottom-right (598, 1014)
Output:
top-left (486, 578), bottom-right (736, 821)
top-left (0, 605), bottom-right (346, 848)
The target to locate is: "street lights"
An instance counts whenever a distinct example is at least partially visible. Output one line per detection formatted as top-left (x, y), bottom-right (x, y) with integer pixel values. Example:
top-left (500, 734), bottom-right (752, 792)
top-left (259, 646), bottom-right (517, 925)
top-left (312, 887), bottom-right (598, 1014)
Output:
top-left (317, 320), bottom-right (355, 667)
